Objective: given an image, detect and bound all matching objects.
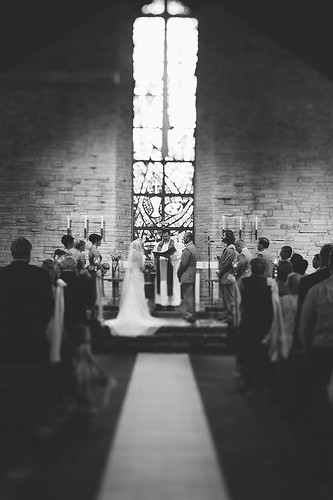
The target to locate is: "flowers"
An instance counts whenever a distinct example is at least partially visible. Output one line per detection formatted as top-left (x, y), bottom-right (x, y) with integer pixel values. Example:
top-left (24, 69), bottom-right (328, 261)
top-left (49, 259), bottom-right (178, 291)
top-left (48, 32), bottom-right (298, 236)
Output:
top-left (102, 263), bottom-right (110, 276)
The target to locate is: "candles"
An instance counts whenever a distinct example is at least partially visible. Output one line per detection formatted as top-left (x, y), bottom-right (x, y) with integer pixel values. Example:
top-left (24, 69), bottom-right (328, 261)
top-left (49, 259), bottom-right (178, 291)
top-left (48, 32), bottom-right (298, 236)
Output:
top-left (255, 217), bottom-right (257, 230)
top-left (222, 216), bottom-right (225, 229)
top-left (68, 215), bottom-right (70, 228)
top-left (239, 217), bottom-right (241, 230)
top-left (101, 216), bottom-right (103, 228)
top-left (84, 216), bottom-right (88, 229)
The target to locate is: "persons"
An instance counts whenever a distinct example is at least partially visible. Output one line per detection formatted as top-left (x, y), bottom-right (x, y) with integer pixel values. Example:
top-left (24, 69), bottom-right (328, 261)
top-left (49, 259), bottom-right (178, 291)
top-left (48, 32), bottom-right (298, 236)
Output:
top-left (298, 249), bottom-right (333, 386)
top-left (103, 238), bottom-right (167, 337)
top-left (0, 236), bottom-right (54, 363)
top-left (73, 240), bottom-right (91, 280)
top-left (277, 243), bottom-right (333, 344)
top-left (57, 235), bottom-right (74, 269)
top-left (89, 233), bottom-right (107, 321)
top-left (232, 257), bottom-right (278, 386)
top-left (256, 237), bottom-right (273, 278)
top-left (151, 228), bottom-right (182, 311)
top-left (235, 240), bottom-right (253, 279)
top-left (217, 230), bottom-right (236, 323)
top-left (177, 232), bottom-right (197, 319)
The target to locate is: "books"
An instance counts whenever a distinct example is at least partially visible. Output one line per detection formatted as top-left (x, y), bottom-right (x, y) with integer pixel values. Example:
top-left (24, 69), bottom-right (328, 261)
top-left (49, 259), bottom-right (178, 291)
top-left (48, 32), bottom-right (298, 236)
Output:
top-left (153, 246), bottom-right (177, 257)
